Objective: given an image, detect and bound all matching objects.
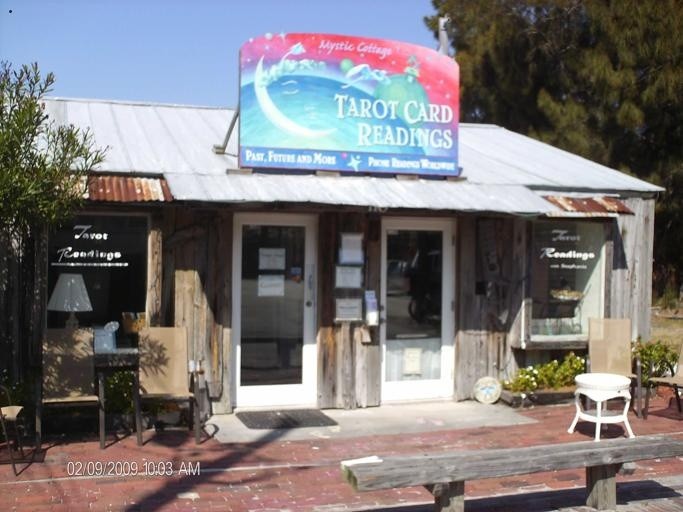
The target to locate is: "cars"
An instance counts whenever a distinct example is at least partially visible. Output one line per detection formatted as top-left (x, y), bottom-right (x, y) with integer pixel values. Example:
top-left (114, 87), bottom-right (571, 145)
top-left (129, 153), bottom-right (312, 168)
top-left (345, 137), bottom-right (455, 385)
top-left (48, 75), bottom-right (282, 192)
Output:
top-left (387, 259), bottom-right (412, 295)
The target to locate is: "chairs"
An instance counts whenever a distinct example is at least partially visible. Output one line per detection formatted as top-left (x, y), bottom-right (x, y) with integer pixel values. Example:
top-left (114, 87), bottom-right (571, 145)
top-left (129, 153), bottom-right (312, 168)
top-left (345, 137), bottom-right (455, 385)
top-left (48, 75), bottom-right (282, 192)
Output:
top-left (0, 328), bottom-right (200, 475)
top-left (588, 319), bottom-right (683, 421)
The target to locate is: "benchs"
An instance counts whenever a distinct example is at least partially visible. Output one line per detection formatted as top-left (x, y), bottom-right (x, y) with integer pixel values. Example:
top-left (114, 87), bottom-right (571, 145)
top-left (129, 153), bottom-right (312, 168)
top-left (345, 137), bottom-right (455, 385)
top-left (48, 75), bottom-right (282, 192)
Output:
top-left (342, 432), bottom-right (683, 512)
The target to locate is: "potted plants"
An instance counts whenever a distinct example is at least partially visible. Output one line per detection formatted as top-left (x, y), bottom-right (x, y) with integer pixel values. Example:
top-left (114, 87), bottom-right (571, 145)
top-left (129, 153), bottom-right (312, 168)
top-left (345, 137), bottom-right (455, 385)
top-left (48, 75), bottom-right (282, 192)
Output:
top-left (499, 335), bottom-right (682, 408)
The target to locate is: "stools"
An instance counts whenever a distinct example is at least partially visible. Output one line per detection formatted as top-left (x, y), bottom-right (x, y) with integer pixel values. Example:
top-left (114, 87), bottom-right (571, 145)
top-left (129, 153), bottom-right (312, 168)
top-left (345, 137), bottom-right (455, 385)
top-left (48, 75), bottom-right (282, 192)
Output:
top-left (568, 373), bottom-right (636, 441)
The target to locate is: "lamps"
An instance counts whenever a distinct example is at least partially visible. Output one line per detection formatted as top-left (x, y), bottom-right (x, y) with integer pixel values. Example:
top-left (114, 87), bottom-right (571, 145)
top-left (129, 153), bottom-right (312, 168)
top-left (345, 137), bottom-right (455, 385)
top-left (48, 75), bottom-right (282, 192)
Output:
top-left (47, 273), bottom-right (94, 328)
